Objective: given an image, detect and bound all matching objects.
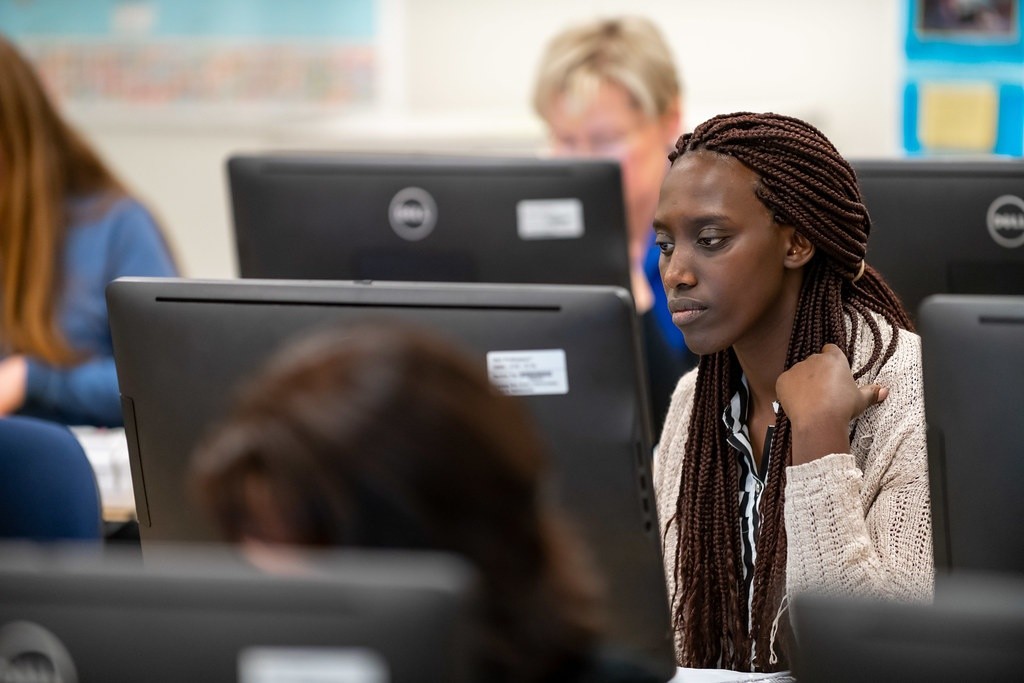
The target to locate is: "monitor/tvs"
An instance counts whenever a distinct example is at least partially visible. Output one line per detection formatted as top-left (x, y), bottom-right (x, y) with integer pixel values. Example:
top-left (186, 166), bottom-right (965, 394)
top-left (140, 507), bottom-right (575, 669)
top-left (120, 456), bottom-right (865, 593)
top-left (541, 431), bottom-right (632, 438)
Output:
top-left (0, 148), bottom-right (1024, 683)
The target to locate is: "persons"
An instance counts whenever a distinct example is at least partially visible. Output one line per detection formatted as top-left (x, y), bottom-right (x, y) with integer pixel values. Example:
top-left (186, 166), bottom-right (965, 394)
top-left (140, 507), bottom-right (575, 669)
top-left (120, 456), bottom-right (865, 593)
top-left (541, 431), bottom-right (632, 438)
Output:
top-left (533, 14), bottom-right (693, 445)
top-left (650, 111), bottom-right (933, 675)
top-left (193, 324), bottom-right (657, 683)
top-left (0, 37), bottom-right (180, 430)
